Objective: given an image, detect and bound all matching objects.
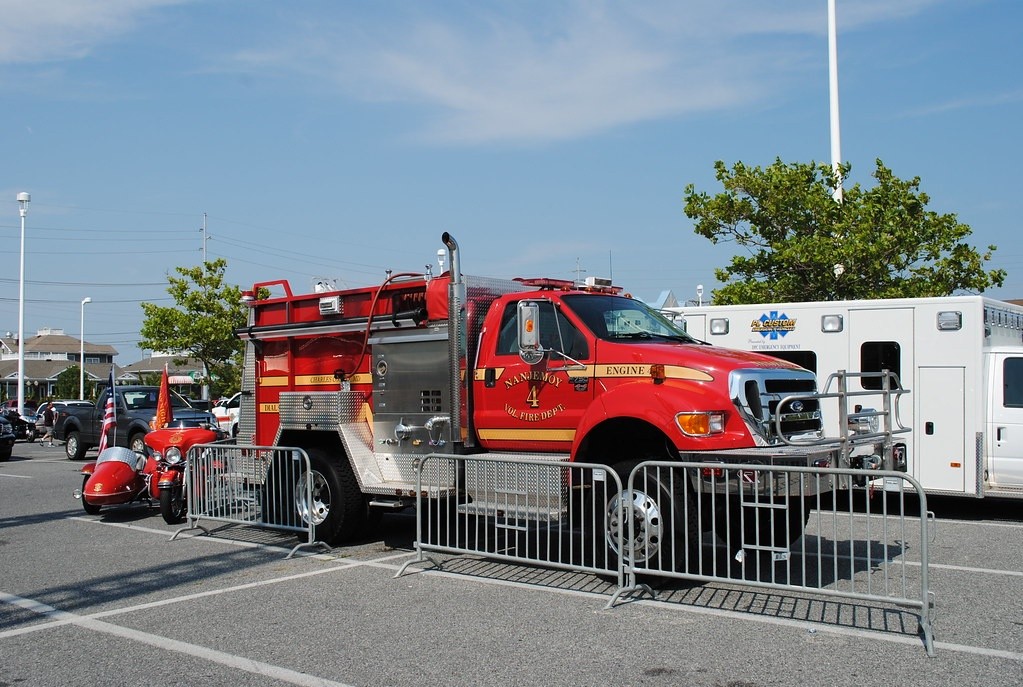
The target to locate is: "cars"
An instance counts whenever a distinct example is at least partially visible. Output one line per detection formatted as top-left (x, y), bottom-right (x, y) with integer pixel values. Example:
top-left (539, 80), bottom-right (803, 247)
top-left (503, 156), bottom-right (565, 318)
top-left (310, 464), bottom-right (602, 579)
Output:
top-left (125, 389), bottom-right (241, 436)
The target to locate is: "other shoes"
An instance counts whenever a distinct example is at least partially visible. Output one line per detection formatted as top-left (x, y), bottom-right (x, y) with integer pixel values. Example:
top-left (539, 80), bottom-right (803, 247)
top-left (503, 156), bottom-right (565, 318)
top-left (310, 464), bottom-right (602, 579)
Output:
top-left (39, 439), bottom-right (44, 446)
top-left (48, 443), bottom-right (56, 447)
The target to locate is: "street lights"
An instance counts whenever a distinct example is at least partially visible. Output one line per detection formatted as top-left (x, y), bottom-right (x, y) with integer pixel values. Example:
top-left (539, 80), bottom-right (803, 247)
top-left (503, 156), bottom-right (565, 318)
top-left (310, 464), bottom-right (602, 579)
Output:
top-left (80, 297), bottom-right (92, 400)
top-left (437, 249), bottom-right (446, 275)
top-left (697, 284), bottom-right (703, 307)
top-left (17, 192), bottom-right (31, 417)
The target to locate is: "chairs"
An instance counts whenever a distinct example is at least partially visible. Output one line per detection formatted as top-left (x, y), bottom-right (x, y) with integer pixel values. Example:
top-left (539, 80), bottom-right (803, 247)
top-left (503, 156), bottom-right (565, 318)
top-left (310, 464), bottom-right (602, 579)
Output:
top-left (130, 449), bottom-right (147, 474)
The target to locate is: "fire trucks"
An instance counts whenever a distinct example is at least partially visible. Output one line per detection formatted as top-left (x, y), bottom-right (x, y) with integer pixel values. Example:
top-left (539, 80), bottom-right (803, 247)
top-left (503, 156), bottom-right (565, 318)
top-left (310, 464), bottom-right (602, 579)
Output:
top-left (225, 232), bottom-right (912, 590)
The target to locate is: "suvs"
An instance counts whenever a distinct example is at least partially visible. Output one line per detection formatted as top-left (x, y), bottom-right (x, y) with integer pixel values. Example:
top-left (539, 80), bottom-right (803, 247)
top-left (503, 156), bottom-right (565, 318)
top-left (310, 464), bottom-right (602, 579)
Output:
top-left (0, 401), bottom-right (94, 462)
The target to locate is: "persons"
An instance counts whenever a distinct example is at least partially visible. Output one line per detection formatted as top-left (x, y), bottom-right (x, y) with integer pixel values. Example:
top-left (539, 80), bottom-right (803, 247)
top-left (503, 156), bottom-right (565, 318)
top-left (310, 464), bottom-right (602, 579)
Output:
top-left (39, 400), bottom-right (57, 447)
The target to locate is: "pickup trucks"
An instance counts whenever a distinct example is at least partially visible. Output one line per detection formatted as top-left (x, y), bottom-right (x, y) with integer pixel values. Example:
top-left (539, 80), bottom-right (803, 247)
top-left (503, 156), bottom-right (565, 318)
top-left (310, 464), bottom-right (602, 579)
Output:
top-left (52, 386), bottom-right (222, 463)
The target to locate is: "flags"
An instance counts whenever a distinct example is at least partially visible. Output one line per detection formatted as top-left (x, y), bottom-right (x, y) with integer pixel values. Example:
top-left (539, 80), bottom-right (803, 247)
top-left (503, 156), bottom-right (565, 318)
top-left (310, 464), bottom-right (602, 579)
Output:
top-left (98, 365), bottom-right (117, 455)
top-left (155, 365), bottom-right (172, 431)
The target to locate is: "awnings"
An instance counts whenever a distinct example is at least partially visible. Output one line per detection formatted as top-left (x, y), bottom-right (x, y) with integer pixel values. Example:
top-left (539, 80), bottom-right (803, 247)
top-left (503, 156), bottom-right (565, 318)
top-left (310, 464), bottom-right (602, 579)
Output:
top-left (168, 376), bottom-right (194, 384)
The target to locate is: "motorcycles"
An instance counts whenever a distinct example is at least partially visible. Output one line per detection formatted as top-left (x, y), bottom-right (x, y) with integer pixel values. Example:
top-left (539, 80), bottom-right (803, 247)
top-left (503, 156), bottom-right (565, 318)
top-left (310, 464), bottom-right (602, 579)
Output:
top-left (78, 426), bottom-right (230, 524)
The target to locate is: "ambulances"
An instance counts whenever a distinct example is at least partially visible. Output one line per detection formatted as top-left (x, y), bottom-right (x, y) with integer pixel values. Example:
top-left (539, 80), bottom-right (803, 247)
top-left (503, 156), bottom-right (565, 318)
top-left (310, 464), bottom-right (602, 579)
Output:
top-left (616, 296), bottom-right (1023, 499)
